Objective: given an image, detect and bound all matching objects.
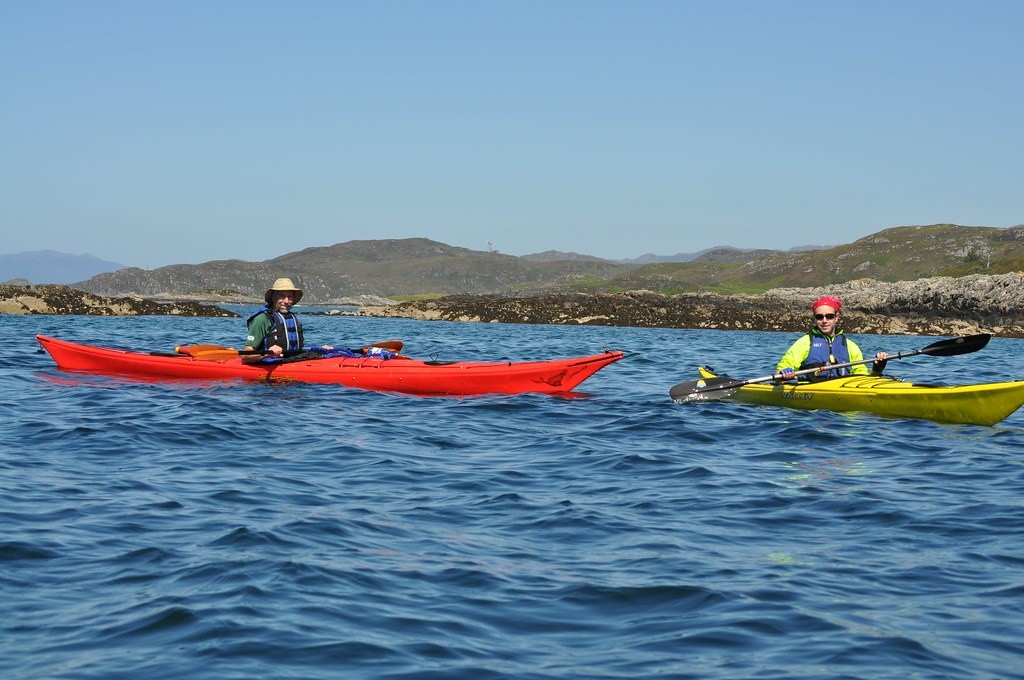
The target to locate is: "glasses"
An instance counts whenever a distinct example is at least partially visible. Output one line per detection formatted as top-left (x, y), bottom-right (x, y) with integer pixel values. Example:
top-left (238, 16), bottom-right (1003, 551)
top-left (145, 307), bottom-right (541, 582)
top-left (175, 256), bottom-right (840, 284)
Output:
top-left (813, 313), bottom-right (836, 320)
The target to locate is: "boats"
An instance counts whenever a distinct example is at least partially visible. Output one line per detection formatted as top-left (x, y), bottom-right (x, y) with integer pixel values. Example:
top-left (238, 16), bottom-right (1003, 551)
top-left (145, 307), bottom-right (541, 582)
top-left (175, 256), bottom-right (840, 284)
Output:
top-left (697, 364), bottom-right (1024, 425)
top-left (36, 298), bottom-right (623, 399)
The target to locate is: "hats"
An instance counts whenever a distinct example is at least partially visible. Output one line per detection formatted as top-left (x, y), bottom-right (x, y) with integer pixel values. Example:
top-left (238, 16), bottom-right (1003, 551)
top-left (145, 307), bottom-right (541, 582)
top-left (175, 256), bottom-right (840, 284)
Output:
top-left (264, 277), bottom-right (304, 305)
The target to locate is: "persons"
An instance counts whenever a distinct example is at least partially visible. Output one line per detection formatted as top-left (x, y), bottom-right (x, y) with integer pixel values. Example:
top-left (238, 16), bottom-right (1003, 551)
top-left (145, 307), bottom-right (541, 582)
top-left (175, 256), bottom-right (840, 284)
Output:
top-left (240, 277), bottom-right (334, 365)
top-left (773, 295), bottom-right (888, 384)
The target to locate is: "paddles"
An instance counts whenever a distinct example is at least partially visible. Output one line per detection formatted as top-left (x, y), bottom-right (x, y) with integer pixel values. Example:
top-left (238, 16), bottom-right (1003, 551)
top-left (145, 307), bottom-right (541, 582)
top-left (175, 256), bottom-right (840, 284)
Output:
top-left (188, 340), bottom-right (405, 360)
top-left (668, 332), bottom-right (991, 402)
